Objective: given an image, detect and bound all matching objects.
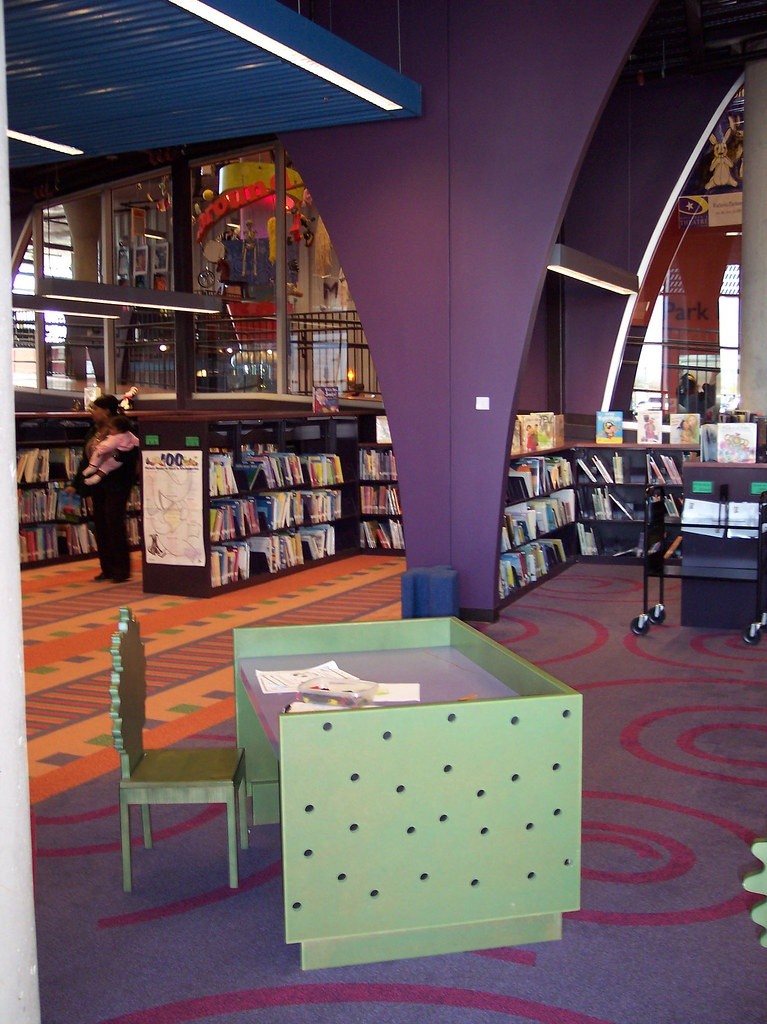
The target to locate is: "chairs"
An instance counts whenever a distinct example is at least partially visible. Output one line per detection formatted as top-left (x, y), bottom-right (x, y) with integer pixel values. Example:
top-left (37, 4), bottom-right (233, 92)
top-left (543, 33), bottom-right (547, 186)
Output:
top-left (106, 602), bottom-right (252, 893)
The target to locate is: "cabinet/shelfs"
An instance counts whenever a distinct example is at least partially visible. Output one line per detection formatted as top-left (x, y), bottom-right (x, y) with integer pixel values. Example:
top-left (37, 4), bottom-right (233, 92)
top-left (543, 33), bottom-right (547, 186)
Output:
top-left (13, 433), bottom-right (767, 610)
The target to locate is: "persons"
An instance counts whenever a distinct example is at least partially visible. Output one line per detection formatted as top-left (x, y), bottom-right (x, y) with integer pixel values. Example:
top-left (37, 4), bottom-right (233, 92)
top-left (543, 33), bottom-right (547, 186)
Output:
top-left (82, 415), bottom-right (138, 485)
top-left (680, 374), bottom-right (713, 419)
top-left (67, 395), bottom-right (138, 582)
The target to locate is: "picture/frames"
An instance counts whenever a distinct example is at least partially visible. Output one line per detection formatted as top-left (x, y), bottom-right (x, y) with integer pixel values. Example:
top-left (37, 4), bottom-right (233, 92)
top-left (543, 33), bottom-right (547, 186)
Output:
top-left (114, 239), bottom-right (171, 291)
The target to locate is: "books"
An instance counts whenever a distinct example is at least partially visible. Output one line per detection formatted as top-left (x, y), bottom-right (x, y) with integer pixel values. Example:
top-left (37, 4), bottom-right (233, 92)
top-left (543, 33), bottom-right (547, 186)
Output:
top-left (17, 487), bottom-right (141, 523)
top-left (577, 522), bottom-right (682, 559)
top-left (210, 441), bottom-right (344, 589)
top-left (670, 413), bottom-right (700, 445)
top-left (17, 447), bottom-right (83, 484)
top-left (504, 451), bottom-right (699, 504)
top-left (636, 410), bottom-right (663, 445)
top-left (359, 447), bottom-right (405, 548)
top-left (500, 486), bottom-right (683, 553)
top-left (19, 517), bottom-right (141, 565)
top-left (596, 411), bottom-right (623, 444)
top-left (511, 412), bottom-right (565, 453)
top-left (717, 423), bottom-right (757, 464)
top-left (498, 538), bottom-right (566, 600)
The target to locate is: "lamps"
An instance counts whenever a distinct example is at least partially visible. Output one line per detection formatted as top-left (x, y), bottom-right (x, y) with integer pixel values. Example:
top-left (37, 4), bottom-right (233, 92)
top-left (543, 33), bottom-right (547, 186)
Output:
top-left (144, 228), bottom-right (167, 240)
top-left (546, 77), bottom-right (639, 297)
top-left (346, 368), bottom-right (365, 397)
top-left (12, 291), bottom-right (126, 320)
top-left (39, 276), bottom-right (224, 316)
top-left (224, 215), bottom-right (241, 228)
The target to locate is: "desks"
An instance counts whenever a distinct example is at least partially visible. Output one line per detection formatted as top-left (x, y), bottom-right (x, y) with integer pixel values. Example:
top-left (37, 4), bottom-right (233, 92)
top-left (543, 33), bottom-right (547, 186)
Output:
top-left (227, 611), bottom-right (588, 974)
